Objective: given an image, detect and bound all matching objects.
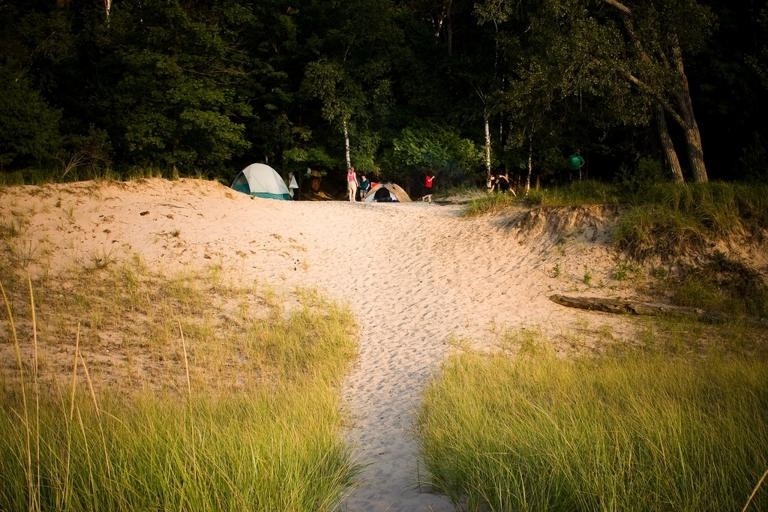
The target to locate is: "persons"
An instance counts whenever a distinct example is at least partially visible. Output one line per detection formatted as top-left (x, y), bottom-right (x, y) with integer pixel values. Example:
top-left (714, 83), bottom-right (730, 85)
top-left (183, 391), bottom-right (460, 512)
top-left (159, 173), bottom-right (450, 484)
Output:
top-left (287, 169), bottom-right (298, 199)
top-left (421, 169), bottom-right (436, 204)
top-left (489, 173), bottom-right (517, 198)
top-left (359, 172), bottom-right (370, 199)
top-left (567, 148), bottom-right (584, 182)
top-left (504, 172), bottom-right (513, 186)
top-left (346, 165), bottom-right (359, 201)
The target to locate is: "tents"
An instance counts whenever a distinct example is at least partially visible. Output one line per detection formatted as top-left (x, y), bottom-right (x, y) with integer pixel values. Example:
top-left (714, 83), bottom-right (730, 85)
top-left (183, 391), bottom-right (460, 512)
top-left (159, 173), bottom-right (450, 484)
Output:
top-left (228, 162), bottom-right (293, 201)
top-left (360, 180), bottom-right (413, 202)
